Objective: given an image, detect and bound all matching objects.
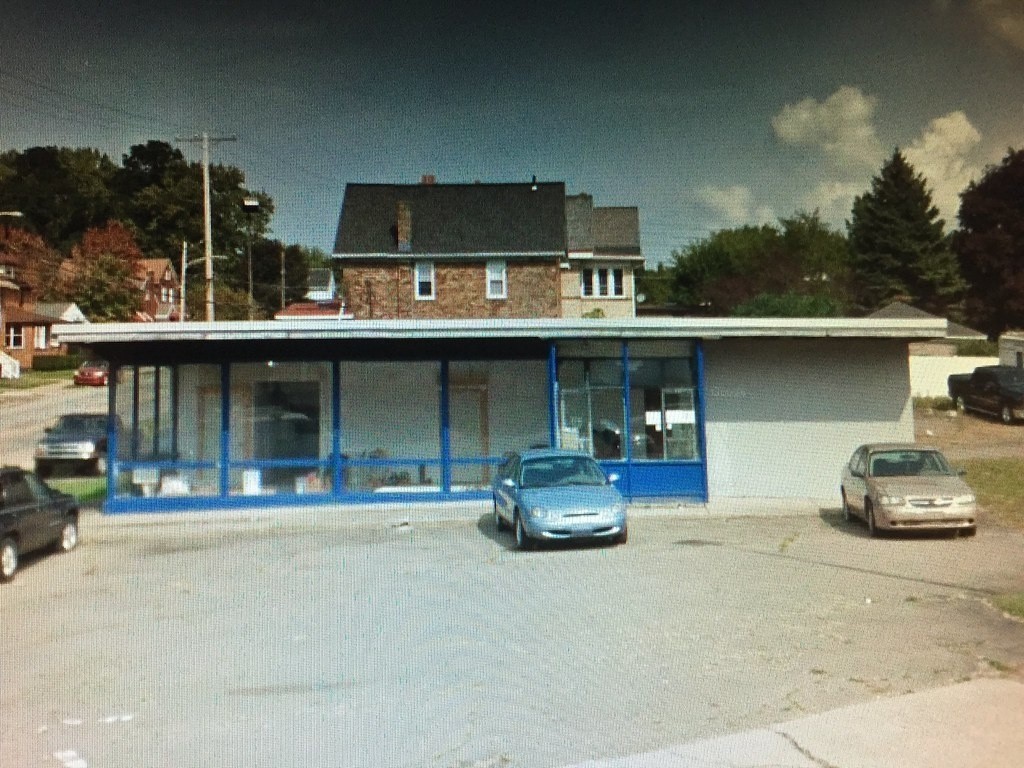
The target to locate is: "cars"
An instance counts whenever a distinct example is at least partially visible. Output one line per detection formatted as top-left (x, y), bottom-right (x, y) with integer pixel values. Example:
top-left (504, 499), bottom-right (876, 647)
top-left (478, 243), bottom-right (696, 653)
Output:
top-left (226, 406), bottom-right (314, 448)
top-left (73, 359), bottom-right (125, 386)
top-left (840, 444), bottom-right (977, 536)
top-left (491, 451), bottom-right (629, 548)
top-left (589, 418), bottom-right (654, 456)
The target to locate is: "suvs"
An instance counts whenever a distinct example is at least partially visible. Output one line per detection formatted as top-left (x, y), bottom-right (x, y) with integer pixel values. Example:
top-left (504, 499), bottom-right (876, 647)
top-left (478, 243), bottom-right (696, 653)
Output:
top-left (0, 465), bottom-right (81, 581)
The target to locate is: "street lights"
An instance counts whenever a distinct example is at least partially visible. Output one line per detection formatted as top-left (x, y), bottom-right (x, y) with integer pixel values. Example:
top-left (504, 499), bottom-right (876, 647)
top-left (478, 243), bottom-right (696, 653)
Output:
top-left (177, 241), bottom-right (227, 322)
top-left (242, 195), bottom-right (261, 319)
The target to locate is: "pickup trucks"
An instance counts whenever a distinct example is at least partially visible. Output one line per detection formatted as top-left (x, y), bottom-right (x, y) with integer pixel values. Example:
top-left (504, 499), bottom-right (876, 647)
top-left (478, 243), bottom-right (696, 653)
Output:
top-left (947, 365), bottom-right (1024, 423)
top-left (33, 412), bottom-right (142, 476)
